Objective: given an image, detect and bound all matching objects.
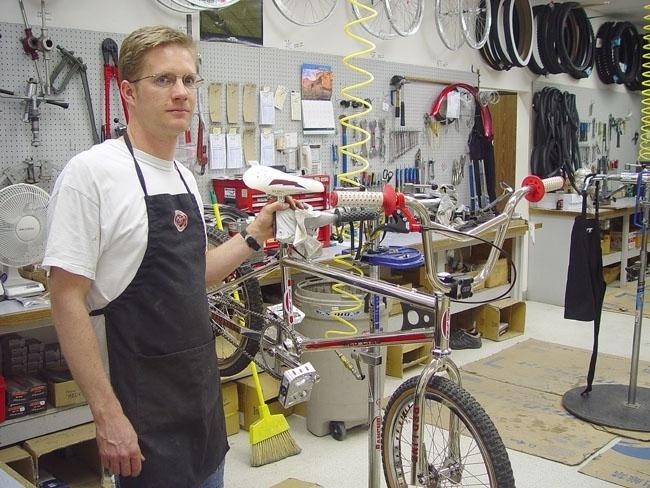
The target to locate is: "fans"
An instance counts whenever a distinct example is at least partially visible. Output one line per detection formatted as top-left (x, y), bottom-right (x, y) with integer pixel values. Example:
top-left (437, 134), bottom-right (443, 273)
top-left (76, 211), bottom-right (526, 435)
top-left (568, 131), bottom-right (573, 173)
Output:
top-left (0, 182), bottom-right (51, 298)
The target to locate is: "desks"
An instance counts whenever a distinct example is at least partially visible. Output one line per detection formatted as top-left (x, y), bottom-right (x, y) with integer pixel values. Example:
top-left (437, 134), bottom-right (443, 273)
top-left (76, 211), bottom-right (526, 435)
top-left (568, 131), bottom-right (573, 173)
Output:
top-left (260, 217), bottom-right (529, 407)
top-left (0, 271), bottom-right (100, 447)
top-left (526, 196), bottom-right (649, 307)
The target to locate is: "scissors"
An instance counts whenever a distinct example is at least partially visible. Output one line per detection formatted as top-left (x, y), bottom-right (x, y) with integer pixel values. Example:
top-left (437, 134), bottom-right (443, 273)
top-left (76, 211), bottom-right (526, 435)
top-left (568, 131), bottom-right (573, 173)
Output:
top-left (382, 169), bottom-right (393, 182)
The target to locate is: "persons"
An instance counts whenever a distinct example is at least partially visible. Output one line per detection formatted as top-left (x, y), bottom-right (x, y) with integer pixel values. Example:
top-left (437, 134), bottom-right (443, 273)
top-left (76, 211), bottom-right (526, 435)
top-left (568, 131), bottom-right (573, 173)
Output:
top-left (40, 21), bottom-right (312, 488)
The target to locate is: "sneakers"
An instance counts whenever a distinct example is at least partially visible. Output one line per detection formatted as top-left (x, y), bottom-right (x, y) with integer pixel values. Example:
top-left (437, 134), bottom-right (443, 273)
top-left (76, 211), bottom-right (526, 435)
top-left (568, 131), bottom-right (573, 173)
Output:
top-left (449, 327), bottom-right (481, 349)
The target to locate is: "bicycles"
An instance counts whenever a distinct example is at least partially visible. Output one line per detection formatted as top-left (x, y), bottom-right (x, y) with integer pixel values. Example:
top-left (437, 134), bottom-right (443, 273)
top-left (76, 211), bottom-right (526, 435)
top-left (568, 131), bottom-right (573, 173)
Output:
top-left (200, 173), bottom-right (567, 488)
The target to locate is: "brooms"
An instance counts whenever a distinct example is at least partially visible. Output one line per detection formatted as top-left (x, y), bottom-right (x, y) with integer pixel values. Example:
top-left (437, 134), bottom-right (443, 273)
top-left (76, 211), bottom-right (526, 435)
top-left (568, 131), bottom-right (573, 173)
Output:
top-left (208, 188), bottom-right (302, 466)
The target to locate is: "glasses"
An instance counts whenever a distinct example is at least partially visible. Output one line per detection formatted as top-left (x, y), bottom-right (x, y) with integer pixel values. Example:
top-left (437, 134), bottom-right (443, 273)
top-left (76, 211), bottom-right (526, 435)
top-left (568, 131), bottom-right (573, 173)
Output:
top-left (129, 72), bottom-right (203, 88)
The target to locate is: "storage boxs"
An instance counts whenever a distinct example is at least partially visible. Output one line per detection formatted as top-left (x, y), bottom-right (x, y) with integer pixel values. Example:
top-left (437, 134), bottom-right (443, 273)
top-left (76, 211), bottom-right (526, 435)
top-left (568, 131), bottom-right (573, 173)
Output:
top-left (237, 367), bottom-right (294, 432)
top-left (220, 382), bottom-right (239, 435)
top-left (1, 422), bottom-right (114, 488)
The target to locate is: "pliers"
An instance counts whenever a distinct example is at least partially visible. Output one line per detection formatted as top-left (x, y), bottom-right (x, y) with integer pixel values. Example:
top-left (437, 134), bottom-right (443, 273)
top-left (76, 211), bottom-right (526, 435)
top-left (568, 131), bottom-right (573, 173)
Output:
top-left (102, 38), bottom-right (128, 138)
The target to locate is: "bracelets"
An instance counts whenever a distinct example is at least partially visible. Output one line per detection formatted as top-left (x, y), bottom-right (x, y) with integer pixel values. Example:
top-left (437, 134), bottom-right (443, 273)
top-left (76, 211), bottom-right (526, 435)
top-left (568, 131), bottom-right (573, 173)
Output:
top-left (239, 229), bottom-right (263, 254)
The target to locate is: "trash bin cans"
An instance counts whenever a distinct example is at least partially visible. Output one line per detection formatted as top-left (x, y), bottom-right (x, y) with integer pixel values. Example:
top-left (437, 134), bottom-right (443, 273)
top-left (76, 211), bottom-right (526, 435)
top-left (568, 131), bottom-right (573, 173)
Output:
top-left (293, 278), bottom-right (391, 441)
